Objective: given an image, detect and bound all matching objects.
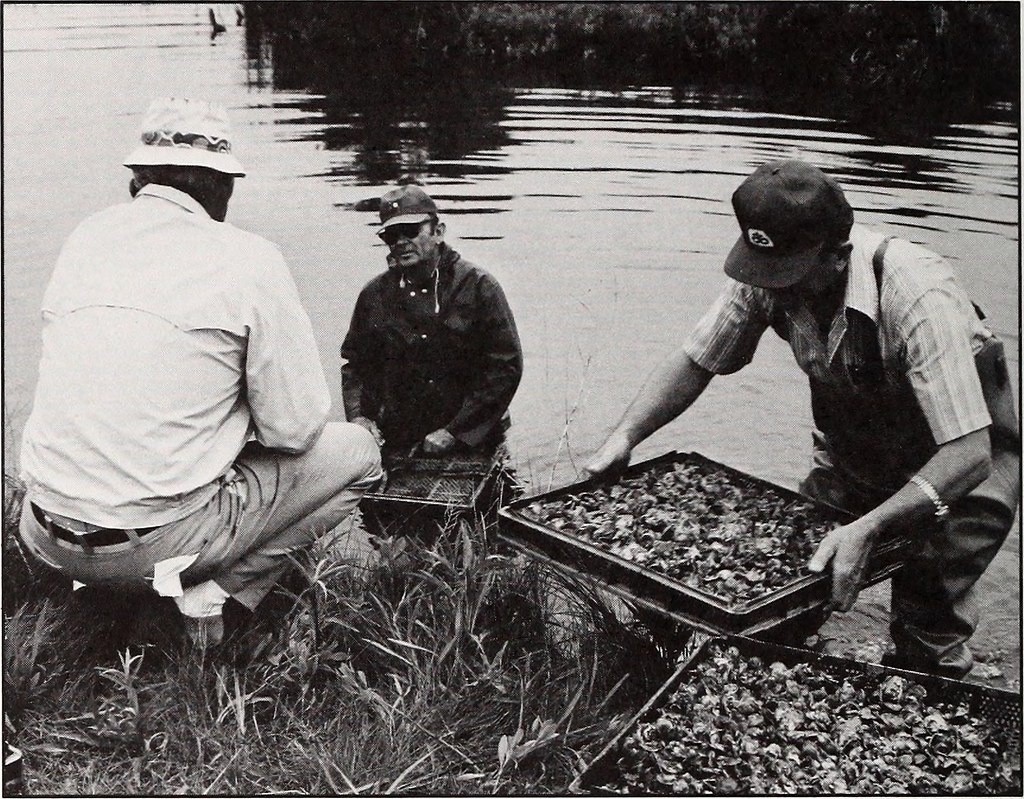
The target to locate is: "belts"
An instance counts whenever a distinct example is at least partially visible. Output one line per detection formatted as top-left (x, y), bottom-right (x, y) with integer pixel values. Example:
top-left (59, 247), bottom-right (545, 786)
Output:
top-left (30, 500), bottom-right (160, 547)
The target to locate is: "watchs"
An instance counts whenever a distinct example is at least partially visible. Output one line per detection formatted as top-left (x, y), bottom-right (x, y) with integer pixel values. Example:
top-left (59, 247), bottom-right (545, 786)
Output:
top-left (911, 472), bottom-right (950, 524)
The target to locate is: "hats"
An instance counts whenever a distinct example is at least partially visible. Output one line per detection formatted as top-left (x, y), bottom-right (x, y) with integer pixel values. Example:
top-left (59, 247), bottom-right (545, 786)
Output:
top-left (723, 159), bottom-right (845, 289)
top-left (123, 98), bottom-right (245, 178)
top-left (374, 184), bottom-right (438, 235)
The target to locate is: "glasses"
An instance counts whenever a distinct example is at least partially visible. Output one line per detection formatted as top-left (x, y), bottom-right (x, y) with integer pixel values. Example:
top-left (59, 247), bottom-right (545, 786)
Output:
top-left (379, 219), bottom-right (432, 244)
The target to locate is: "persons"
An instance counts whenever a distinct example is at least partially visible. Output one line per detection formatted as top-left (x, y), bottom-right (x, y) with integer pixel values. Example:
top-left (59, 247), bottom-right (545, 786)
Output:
top-left (343, 183), bottom-right (525, 461)
top-left (20, 99), bottom-right (385, 661)
top-left (571, 159), bottom-right (1019, 686)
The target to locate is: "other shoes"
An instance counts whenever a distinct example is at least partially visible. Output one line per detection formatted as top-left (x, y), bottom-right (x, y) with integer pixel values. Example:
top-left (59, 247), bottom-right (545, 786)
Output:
top-left (129, 596), bottom-right (251, 676)
